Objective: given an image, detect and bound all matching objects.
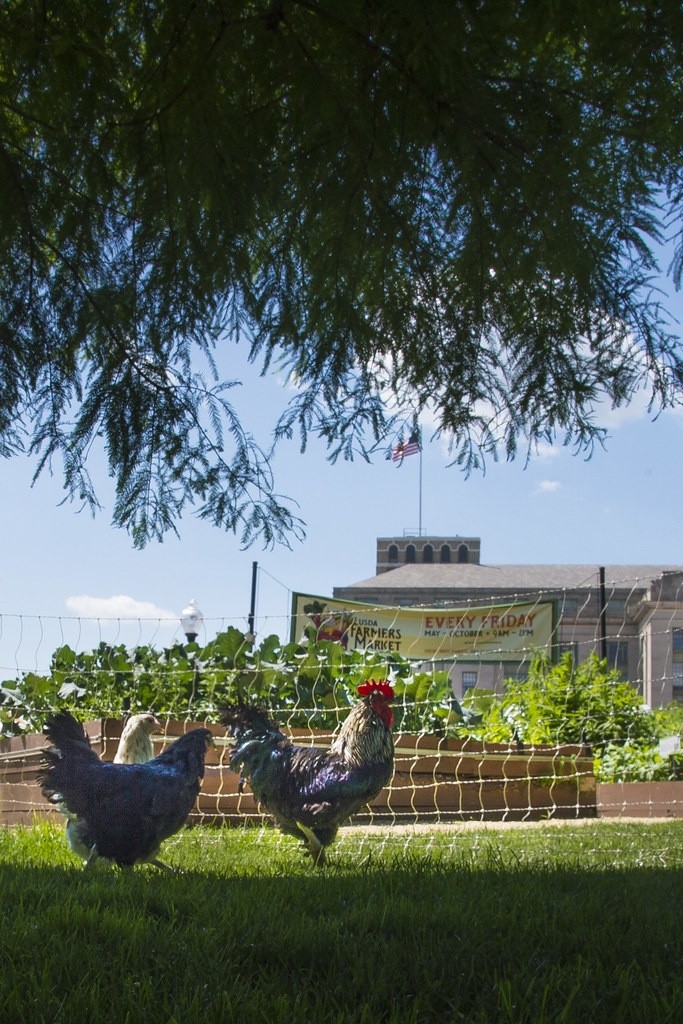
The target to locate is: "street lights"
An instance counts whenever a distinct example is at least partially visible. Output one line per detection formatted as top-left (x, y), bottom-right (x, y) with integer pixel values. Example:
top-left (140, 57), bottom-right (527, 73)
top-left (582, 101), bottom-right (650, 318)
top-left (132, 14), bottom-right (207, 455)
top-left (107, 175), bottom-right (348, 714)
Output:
top-left (180, 599), bottom-right (204, 645)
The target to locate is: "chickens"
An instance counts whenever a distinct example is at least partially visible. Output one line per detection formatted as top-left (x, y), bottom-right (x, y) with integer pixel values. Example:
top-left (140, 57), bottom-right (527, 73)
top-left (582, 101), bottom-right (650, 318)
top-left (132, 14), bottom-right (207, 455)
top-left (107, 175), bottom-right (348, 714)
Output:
top-left (40, 711), bottom-right (183, 878)
top-left (212, 676), bottom-right (398, 870)
top-left (34, 705), bottom-right (218, 883)
